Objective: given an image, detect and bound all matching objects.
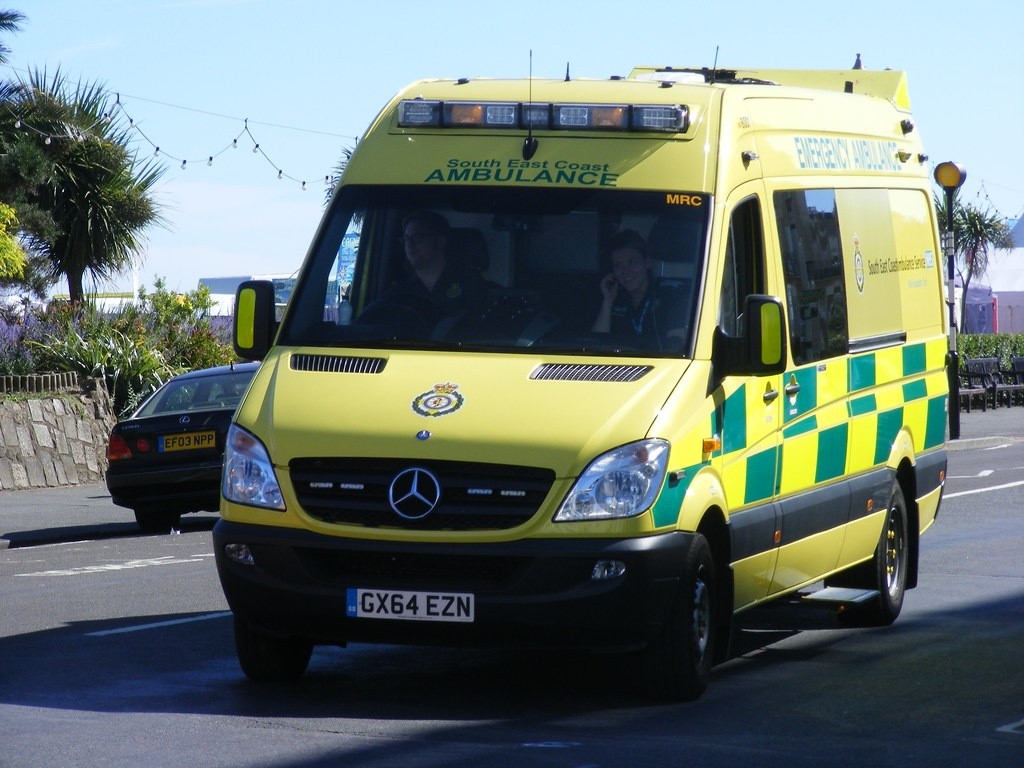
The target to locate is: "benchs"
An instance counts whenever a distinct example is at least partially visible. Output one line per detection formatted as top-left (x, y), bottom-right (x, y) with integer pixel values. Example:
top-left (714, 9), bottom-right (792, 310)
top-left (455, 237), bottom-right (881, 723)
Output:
top-left (957, 353), bottom-right (1024, 413)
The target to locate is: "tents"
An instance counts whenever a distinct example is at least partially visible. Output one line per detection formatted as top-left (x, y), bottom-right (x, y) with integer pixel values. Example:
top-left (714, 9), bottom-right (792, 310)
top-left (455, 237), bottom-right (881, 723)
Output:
top-left (945, 279), bottom-right (994, 335)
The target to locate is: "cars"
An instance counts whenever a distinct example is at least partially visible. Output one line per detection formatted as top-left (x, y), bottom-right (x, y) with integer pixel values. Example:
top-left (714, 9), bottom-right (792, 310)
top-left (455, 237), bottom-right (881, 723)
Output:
top-left (103, 362), bottom-right (266, 535)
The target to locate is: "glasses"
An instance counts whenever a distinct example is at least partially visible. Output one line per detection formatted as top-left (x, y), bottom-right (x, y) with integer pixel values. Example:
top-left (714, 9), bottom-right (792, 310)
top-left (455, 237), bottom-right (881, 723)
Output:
top-left (399, 232), bottom-right (438, 246)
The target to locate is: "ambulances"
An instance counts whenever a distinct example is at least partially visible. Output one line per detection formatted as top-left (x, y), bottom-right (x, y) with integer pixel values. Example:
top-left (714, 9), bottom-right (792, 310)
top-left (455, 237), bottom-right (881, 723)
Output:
top-left (203, 41), bottom-right (950, 710)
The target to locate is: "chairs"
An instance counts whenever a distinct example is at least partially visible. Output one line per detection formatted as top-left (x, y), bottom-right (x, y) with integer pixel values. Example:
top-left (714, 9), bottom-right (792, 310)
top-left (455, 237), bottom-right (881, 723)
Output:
top-left (446, 228), bottom-right (505, 293)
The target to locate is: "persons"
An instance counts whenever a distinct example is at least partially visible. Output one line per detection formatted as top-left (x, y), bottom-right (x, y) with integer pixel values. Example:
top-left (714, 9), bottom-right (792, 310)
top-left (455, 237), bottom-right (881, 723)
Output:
top-left (347, 209), bottom-right (485, 343)
top-left (579, 229), bottom-right (690, 346)
